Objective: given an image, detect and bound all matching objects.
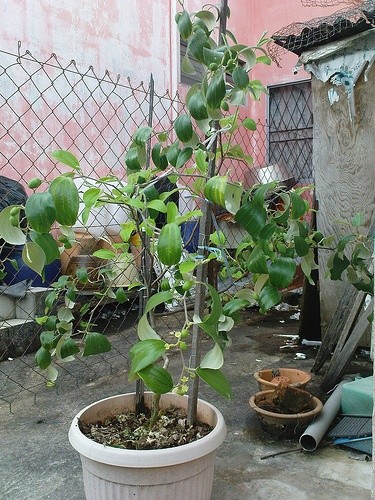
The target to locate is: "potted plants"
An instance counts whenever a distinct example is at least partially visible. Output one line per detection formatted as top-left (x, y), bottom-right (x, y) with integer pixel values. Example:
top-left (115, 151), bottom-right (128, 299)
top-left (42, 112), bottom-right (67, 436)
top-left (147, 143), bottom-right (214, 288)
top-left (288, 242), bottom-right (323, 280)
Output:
top-left (0, 1), bottom-right (373, 500)
top-left (252, 368), bottom-right (311, 392)
top-left (248, 387), bottom-right (324, 439)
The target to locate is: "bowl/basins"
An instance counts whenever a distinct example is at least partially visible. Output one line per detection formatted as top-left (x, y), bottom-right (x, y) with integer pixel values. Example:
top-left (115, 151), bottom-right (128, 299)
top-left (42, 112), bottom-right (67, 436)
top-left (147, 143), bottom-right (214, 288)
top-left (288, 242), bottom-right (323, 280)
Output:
top-left (241, 161), bottom-right (295, 190)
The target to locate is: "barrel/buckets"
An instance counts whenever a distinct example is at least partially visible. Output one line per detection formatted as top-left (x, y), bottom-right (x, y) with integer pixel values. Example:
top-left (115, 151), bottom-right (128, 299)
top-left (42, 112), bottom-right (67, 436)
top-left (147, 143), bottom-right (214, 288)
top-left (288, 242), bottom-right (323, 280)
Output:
top-left (180, 221), bottom-right (200, 254)
top-left (0, 233), bottom-right (62, 289)
top-left (104, 252), bottom-right (142, 288)
